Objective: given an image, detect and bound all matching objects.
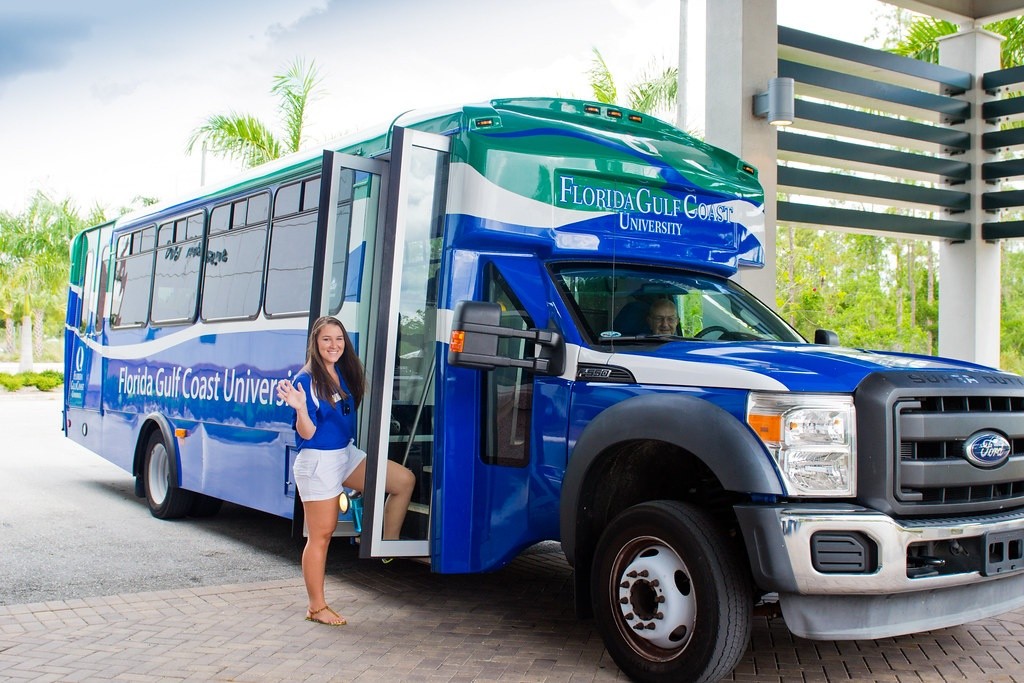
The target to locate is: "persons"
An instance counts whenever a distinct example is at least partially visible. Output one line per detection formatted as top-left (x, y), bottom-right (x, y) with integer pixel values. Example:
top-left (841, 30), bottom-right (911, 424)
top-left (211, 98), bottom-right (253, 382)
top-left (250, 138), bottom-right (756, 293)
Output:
top-left (277, 316), bottom-right (416, 625)
top-left (648, 300), bottom-right (678, 338)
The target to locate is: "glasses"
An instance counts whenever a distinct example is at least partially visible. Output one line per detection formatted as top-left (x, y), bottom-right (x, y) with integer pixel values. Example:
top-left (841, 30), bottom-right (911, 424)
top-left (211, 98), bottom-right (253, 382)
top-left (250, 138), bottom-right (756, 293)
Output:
top-left (340, 391), bottom-right (351, 416)
top-left (650, 316), bottom-right (679, 323)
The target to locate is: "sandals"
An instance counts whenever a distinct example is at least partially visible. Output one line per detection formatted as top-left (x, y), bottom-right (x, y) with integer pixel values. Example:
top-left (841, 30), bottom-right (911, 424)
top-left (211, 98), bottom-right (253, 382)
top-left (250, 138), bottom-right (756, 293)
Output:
top-left (305, 605), bottom-right (346, 626)
top-left (382, 555), bottom-right (395, 564)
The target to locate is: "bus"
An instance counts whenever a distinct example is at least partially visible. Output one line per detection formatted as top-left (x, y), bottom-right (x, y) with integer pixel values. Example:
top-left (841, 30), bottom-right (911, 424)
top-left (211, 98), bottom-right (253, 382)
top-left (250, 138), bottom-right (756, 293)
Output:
top-left (59, 95), bottom-right (1024, 683)
